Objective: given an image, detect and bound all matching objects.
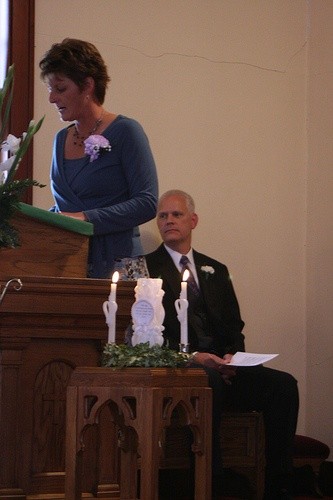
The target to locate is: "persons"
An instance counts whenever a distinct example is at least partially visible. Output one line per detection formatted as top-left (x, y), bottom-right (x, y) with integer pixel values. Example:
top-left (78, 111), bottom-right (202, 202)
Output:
top-left (39, 39), bottom-right (159, 280)
top-left (137, 191), bottom-right (301, 500)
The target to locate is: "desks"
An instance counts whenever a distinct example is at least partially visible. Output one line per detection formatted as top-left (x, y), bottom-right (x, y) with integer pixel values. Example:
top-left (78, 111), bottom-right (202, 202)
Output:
top-left (64, 367), bottom-right (212, 500)
top-left (0, 274), bottom-right (137, 500)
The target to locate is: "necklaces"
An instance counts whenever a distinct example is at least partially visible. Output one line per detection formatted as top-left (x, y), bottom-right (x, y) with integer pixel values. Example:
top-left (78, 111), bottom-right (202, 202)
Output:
top-left (72, 109), bottom-right (105, 146)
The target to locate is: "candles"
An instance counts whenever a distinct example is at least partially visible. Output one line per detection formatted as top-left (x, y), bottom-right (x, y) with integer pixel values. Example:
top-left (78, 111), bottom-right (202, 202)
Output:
top-left (130, 278), bottom-right (166, 349)
top-left (102, 271), bottom-right (119, 345)
top-left (174, 270), bottom-right (190, 345)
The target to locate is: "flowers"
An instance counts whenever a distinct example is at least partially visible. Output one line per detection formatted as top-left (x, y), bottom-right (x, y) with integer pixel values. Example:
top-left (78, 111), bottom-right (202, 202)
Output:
top-left (83, 134), bottom-right (112, 163)
top-left (0, 64), bottom-right (47, 249)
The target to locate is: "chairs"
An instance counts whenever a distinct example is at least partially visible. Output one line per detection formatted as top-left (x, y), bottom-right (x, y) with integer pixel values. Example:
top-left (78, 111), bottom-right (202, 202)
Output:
top-left (137, 408), bottom-right (266, 500)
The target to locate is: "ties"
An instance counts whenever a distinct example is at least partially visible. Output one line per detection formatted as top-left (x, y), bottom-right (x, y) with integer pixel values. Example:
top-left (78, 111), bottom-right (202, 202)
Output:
top-left (179, 256), bottom-right (201, 300)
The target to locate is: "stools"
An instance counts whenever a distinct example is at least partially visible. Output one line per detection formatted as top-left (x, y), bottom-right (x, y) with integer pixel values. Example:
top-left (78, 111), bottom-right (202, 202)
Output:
top-left (291, 435), bottom-right (330, 500)
